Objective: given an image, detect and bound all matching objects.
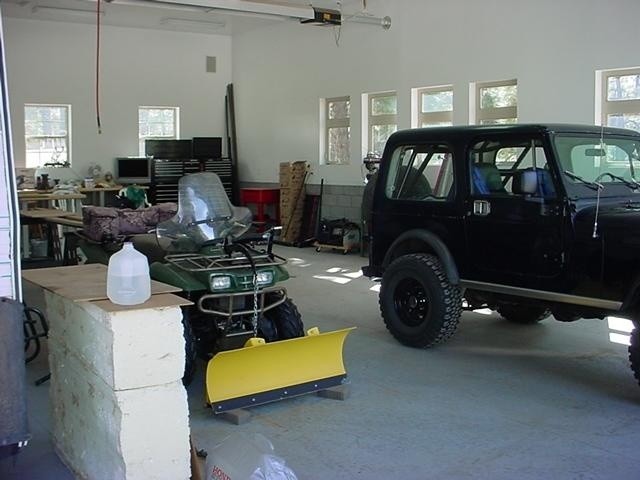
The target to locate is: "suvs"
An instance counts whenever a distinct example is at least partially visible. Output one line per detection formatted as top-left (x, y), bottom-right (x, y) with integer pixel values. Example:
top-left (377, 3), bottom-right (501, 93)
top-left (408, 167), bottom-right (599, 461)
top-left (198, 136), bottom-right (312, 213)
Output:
top-left (359, 123), bottom-right (639, 383)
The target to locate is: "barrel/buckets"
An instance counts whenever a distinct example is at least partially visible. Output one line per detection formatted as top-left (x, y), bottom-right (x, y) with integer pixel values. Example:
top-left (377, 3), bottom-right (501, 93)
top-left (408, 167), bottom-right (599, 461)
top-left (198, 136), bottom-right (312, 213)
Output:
top-left (84, 178), bottom-right (95, 188)
top-left (31, 238), bottom-right (48, 257)
top-left (106, 242), bottom-right (151, 306)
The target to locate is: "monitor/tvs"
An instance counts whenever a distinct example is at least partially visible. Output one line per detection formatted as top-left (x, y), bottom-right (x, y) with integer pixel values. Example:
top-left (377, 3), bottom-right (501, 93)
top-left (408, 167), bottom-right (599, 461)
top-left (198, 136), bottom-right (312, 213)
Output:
top-left (114, 156), bottom-right (151, 189)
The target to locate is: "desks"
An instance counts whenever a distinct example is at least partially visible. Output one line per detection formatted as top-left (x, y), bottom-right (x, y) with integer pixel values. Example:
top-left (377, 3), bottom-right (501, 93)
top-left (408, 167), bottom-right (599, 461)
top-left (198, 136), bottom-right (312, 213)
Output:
top-left (17, 186), bottom-right (150, 263)
top-left (240, 188), bottom-right (280, 233)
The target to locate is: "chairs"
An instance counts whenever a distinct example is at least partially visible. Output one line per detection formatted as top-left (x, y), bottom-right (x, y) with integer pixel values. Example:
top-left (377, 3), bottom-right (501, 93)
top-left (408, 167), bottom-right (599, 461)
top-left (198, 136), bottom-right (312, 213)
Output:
top-left (391, 162), bottom-right (538, 198)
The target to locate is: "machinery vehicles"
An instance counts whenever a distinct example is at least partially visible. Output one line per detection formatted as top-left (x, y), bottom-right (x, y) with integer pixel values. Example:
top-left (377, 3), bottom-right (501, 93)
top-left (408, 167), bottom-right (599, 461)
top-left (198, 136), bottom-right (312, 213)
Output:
top-left (63, 173), bottom-right (358, 415)
